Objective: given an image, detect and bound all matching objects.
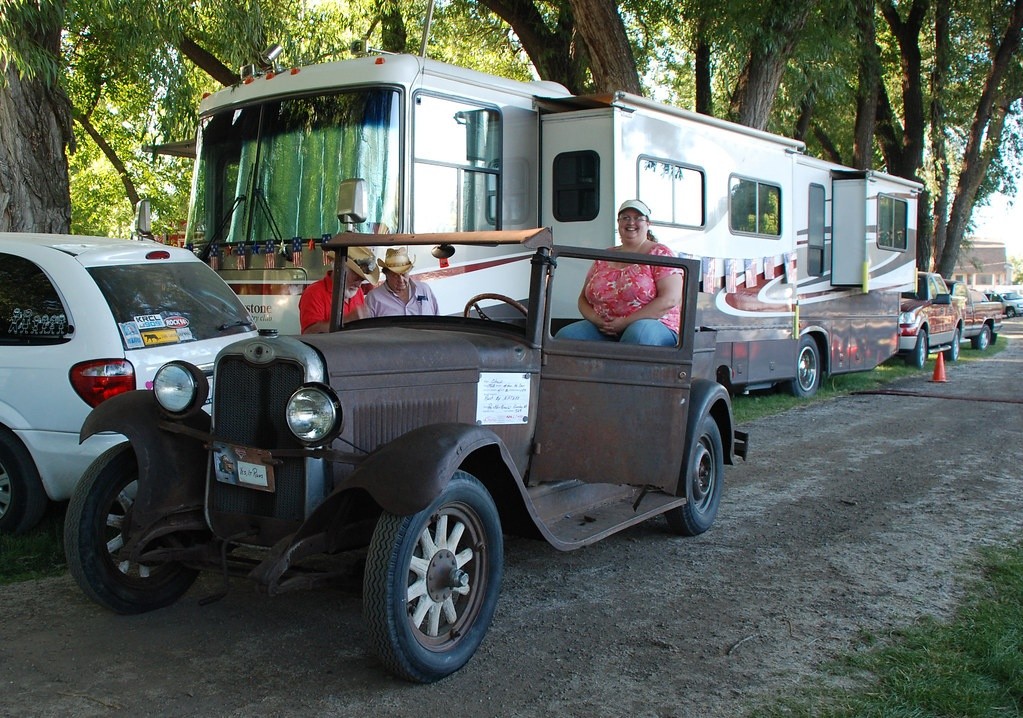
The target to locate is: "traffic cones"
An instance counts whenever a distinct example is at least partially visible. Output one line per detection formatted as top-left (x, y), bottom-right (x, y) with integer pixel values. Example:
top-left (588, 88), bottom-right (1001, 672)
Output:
top-left (928, 351), bottom-right (954, 383)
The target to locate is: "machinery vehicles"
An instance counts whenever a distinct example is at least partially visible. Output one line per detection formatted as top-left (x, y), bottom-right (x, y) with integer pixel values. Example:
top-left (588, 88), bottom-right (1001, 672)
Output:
top-left (60, 206), bottom-right (747, 687)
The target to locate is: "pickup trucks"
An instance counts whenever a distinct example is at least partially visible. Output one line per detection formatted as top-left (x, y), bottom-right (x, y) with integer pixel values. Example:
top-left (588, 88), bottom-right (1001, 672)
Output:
top-left (958, 285), bottom-right (1005, 352)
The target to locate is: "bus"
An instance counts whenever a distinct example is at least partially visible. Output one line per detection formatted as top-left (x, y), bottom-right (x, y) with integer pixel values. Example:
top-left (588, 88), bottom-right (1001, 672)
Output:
top-left (132, 5), bottom-right (924, 413)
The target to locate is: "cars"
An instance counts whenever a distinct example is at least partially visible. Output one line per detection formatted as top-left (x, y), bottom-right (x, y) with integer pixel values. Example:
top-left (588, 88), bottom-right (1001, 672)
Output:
top-left (0, 224), bottom-right (270, 541)
top-left (893, 261), bottom-right (965, 368)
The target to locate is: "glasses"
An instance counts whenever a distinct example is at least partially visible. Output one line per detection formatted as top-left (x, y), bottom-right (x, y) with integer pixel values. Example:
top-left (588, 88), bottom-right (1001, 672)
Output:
top-left (620, 214), bottom-right (647, 222)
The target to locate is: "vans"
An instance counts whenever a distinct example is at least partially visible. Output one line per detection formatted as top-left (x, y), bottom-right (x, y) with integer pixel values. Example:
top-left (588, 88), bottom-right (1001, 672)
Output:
top-left (982, 291), bottom-right (1023, 319)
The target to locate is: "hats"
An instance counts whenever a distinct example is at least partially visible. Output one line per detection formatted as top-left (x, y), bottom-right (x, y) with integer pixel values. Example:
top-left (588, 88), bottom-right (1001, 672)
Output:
top-left (328, 246), bottom-right (379, 286)
top-left (619, 199), bottom-right (651, 221)
top-left (376, 247), bottom-right (416, 274)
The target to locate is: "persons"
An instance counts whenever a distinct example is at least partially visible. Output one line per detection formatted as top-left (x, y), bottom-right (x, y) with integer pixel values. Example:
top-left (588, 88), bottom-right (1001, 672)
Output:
top-left (298, 247), bottom-right (379, 336)
top-left (553, 199), bottom-right (684, 347)
top-left (364, 246), bottom-right (441, 316)
